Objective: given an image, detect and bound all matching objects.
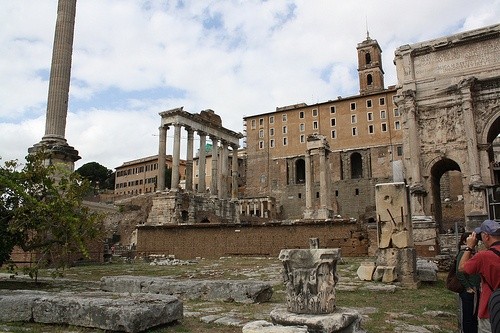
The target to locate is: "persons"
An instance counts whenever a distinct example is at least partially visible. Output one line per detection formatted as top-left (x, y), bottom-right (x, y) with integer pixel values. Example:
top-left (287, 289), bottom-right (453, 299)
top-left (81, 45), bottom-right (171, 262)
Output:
top-left (111, 244), bottom-right (115, 254)
top-left (458, 220), bottom-right (500, 333)
top-left (456, 232), bottom-right (481, 333)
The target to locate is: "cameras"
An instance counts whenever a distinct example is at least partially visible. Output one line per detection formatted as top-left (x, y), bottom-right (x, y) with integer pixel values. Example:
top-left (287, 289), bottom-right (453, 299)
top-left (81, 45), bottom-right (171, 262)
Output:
top-left (476, 232), bottom-right (482, 241)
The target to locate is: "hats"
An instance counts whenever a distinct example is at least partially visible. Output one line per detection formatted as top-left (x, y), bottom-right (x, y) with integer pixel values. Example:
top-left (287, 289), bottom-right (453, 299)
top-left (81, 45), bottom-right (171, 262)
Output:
top-left (473, 220), bottom-right (500, 236)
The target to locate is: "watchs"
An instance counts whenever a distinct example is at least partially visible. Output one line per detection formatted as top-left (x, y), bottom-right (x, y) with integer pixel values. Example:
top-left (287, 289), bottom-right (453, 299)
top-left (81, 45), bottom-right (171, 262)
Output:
top-left (464, 247), bottom-right (473, 252)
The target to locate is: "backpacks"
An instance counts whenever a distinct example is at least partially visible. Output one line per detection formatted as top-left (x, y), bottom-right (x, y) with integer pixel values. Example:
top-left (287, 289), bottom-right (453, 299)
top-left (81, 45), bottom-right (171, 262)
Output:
top-left (446, 250), bottom-right (464, 292)
top-left (480, 248), bottom-right (500, 333)
